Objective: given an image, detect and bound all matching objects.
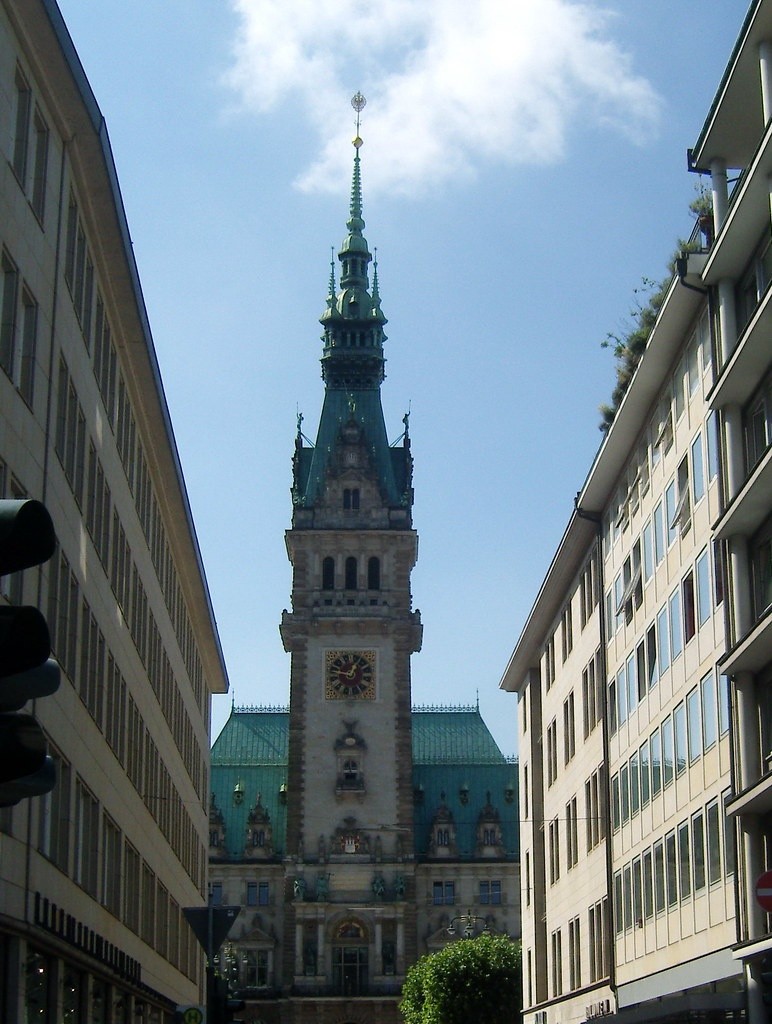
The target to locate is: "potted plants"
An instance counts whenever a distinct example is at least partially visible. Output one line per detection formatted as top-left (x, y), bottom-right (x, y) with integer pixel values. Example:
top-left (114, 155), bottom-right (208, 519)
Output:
top-left (687, 173), bottom-right (713, 236)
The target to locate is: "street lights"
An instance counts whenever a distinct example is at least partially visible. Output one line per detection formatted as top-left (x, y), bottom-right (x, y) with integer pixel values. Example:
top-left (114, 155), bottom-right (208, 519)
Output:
top-left (215, 940), bottom-right (249, 998)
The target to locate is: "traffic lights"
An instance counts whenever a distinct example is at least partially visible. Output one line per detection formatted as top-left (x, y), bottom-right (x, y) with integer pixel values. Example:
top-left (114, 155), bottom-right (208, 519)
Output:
top-left (0, 496), bottom-right (58, 809)
top-left (6, 657), bottom-right (63, 808)
top-left (217, 985), bottom-right (247, 1024)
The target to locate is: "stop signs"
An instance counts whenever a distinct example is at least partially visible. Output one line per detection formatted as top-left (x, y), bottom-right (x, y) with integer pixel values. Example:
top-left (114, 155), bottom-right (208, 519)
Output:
top-left (753, 869), bottom-right (772, 914)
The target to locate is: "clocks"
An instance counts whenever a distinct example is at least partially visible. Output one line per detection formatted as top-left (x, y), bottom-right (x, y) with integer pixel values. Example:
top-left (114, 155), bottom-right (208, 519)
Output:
top-left (321, 646), bottom-right (379, 704)
